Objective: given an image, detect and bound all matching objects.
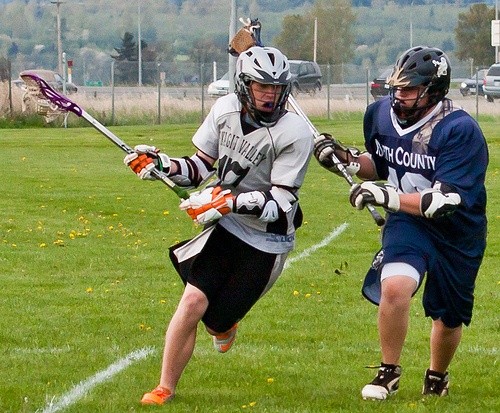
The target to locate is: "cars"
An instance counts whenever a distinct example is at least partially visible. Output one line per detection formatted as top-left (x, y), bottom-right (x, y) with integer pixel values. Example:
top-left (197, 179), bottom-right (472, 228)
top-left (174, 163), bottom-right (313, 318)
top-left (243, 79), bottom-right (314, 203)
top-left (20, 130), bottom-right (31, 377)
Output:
top-left (13, 68), bottom-right (78, 96)
top-left (207, 69), bottom-right (235, 98)
top-left (461, 69), bottom-right (487, 98)
top-left (370, 68), bottom-right (395, 101)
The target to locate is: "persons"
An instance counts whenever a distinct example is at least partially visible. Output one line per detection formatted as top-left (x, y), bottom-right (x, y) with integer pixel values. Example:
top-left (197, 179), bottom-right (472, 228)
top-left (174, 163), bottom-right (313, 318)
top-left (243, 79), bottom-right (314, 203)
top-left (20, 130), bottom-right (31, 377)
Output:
top-left (311, 45), bottom-right (491, 401)
top-left (122, 46), bottom-right (313, 407)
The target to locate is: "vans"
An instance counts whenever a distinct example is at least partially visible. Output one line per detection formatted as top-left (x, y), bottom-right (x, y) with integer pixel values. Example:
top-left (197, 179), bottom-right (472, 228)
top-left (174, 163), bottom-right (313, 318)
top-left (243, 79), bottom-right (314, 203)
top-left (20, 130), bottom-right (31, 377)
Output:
top-left (481, 62), bottom-right (500, 103)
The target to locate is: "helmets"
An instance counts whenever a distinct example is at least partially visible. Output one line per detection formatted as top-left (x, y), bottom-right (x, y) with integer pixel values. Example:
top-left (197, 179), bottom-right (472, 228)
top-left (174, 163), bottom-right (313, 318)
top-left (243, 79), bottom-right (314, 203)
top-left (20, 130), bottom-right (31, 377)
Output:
top-left (232, 46), bottom-right (292, 127)
top-left (388, 45), bottom-right (450, 127)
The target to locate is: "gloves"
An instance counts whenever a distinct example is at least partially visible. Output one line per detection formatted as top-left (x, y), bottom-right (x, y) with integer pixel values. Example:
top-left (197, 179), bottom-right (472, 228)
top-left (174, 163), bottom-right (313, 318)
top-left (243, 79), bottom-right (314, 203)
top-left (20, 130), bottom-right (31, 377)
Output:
top-left (178, 184), bottom-right (234, 224)
top-left (313, 132), bottom-right (360, 178)
top-left (348, 181), bottom-right (400, 214)
top-left (122, 144), bottom-right (171, 181)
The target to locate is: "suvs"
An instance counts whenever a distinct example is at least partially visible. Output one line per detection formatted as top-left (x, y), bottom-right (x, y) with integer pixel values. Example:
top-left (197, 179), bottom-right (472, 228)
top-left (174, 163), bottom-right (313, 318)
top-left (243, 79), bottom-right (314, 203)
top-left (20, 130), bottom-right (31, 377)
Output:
top-left (288, 59), bottom-right (323, 95)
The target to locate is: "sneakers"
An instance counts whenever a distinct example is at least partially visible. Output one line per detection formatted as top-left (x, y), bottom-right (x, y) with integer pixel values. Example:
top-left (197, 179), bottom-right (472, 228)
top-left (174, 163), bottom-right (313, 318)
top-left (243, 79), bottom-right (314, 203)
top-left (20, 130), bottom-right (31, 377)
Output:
top-left (360, 362), bottom-right (403, 400)
top-left (213, 323), bottom-right (238, 353)
top-left (140, 385), bottom-right (176, 406)
top-left (422, 369), bottom-right (450, 399)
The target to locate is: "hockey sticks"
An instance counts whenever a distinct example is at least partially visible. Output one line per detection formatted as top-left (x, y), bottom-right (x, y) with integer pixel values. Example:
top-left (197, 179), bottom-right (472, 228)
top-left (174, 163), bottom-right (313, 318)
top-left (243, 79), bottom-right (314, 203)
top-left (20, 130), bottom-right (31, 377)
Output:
top-left (21, 74), bottom-right (191, 200)
top-left (227, 18), bottom-right (385, 226)
